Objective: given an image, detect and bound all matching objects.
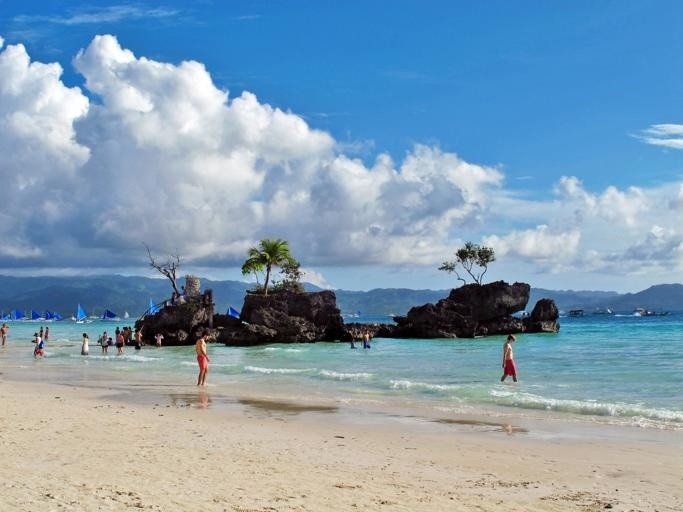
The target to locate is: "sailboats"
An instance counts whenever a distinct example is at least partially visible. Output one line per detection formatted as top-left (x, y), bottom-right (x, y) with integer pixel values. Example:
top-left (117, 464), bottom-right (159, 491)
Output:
top-left (0, 297), bottom-right (160, 323)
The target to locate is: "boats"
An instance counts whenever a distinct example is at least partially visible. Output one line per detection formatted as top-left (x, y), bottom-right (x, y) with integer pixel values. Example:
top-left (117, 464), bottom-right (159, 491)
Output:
top-left (558, 304), bottom-right (671, 318)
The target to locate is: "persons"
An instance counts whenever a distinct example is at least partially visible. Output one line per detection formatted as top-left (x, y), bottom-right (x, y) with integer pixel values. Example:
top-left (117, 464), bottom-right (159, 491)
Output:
top-left (31, 332), bottom-right (43, 360)
top-left (194, 330), bottom-right (210, 386)
top-left (361, 329), bottom-right (370, 350)
top-left (498, 334), bottom-right (518, 384)
top-left (44, 327), bottom-right (48, 340)
top-left (0, 323), bottom-right (9, 347)
top-left (115, 326), bottom-right (143, 354)
top-left (170, 292), bottom-right (177, 307)
top-left (80, 333), bottom-right (89, 356)
top-left (97, 331), bottom-right (109, 354)
top-left (180, 285), bottom-right (185, 295)
top-left (107, 337), bottom-right (113, 346)
top-left (154, 333), bottom-right (164, 348)
